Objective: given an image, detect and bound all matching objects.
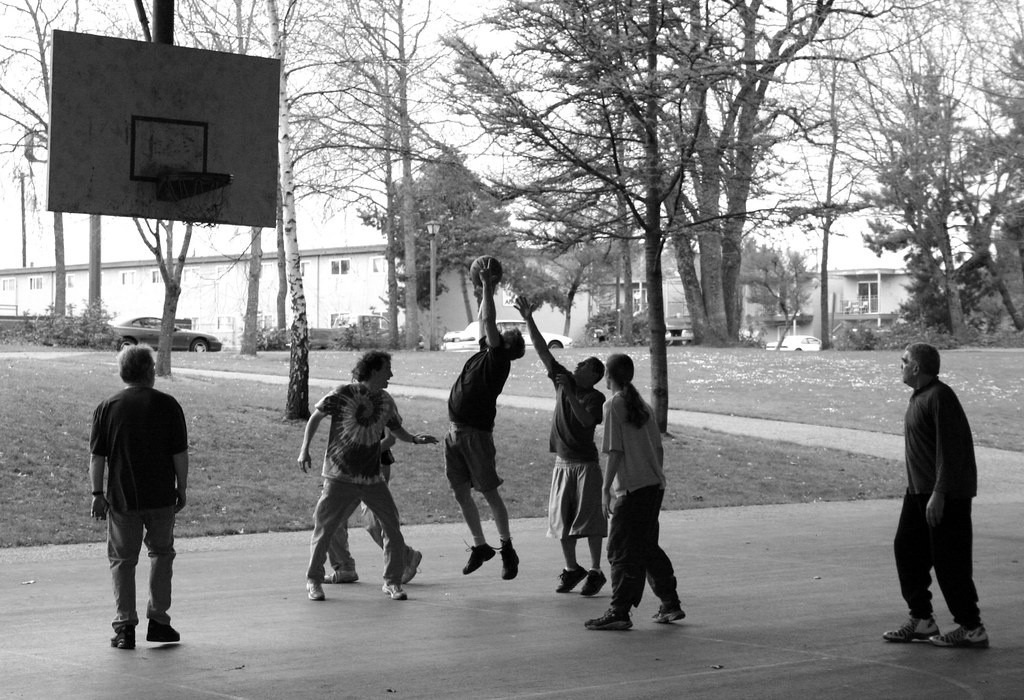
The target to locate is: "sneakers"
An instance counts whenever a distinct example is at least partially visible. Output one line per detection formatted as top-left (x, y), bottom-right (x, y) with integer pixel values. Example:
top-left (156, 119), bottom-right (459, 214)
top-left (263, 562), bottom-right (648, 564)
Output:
top-left (498, 539), bottom-right (519, 580)
top-left (306, 580), bottom-right (325, 601)
top-left (580, 569), bottom-right (607, 595)
top-left (882, 614), bottom-right (941, 643)
top-left (650, 604), bottom-right (686, 622)
top-left (381, 582), bottom-right (408, 600)
top-left (584, 608), bottom-right (633, 630)
top-left (929, 622), bottom-right (989, 649)
top-left (462, 543), bottom-right (496, 575)
top-left (554, 565), bottom-right (588, 593)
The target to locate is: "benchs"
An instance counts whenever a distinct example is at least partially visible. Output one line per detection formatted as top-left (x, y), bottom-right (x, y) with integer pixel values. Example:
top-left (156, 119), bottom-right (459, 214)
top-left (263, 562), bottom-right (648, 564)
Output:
top-left (664, 337), bottom-right (692, 341)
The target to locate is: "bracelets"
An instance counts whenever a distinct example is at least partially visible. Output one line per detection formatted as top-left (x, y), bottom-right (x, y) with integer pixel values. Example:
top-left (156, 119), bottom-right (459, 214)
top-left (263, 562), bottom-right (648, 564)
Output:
top-left (92, 491), bottom-right (103, 496)
top-left (412, 434), bottom-right (419, 446)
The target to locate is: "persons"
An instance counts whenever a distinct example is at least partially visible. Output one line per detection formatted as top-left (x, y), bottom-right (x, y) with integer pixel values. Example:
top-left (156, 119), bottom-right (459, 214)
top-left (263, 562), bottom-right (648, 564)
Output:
top-left (444, 258), bottom-right (526, 580)
top-left (882, 341), bottom-right (990, 648)
top-left (323, 426), bottom-right (423, 584)
top-left (584, 351), bottom-right (685, 630)
top-left (297, 351), bottom-right (439, 602)
top-left (513, 294), bottom-right (606, 596)
top-left (88, 342), bottom-right (190, 650)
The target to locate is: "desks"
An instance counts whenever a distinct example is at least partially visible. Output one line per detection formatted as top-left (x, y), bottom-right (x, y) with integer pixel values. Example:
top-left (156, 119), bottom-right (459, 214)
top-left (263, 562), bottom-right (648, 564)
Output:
top-left (667, 327), bottom-right (690, 345)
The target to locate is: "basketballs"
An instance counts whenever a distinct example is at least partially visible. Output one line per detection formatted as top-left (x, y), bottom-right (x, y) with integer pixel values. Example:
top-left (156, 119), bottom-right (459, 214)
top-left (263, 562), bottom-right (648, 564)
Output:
top-left (470, 254), bottom-right (502, 288)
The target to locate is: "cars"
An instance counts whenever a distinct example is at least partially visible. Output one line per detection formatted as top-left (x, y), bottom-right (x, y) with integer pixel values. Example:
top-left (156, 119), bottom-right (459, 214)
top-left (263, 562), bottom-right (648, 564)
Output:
top-left (765, 335), bottom-right (822, 352)
top-left (439, 319), bottom-right (573, 352)
top-left (106, 316), bottom-right (223, 354)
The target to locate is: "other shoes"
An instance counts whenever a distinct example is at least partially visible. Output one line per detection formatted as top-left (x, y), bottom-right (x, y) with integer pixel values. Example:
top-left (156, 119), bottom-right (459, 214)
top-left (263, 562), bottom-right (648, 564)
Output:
top-left (110, 626), bottom-right (136, 649)
top-left (322, 568), bottom-right (359, 583)
top-left (401, 551), bottom-right (422, 583)
top-left (146, 621), bottom-right (181, 642)
